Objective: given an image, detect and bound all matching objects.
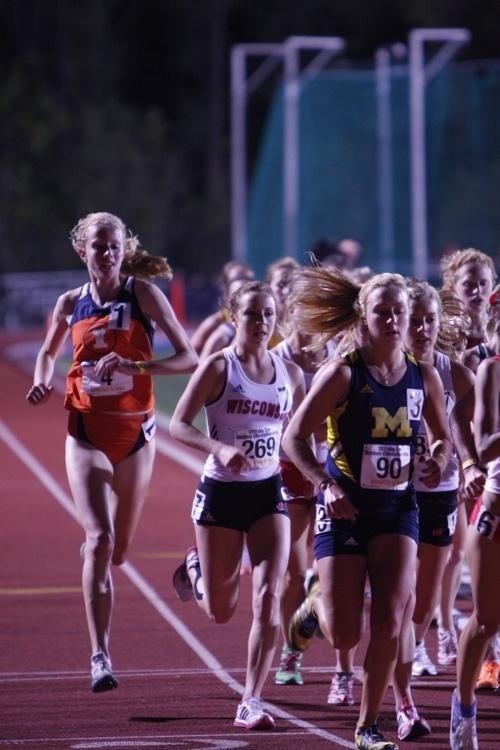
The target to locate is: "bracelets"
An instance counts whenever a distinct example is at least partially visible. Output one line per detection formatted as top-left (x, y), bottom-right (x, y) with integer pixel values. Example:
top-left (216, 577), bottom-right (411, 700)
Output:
top-left (134, 355), bottom-right (148, 377)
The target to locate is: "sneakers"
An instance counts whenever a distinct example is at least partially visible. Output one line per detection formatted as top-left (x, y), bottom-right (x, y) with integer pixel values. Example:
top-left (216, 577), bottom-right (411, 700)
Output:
top-left (173, 546), bottom-right (198, 601)
top-left (450, 688), bottom-right (478, 750)
top-left (233, 699), bottom-right (276, 731)
top-left (90, 652), bottom-right (118, 692)
top-left (354, 721), bottom-right (399, 750)
top-left (411, 628), bottom-right (499, 690)
top-left (327, 672), bottom-right (355, 706)
top-left (275, 647), bottom-right (303, 685)
top-left (288, 580), bottom-right (317, 652)
top-left (397, 707), bottom-right (432, 742)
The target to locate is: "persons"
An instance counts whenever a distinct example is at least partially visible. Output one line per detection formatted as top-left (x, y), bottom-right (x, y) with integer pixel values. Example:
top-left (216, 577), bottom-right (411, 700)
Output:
top-left (25, 206), bottom-right (202, 695)
top-left (168, 234), bottom-right (500, 750)
